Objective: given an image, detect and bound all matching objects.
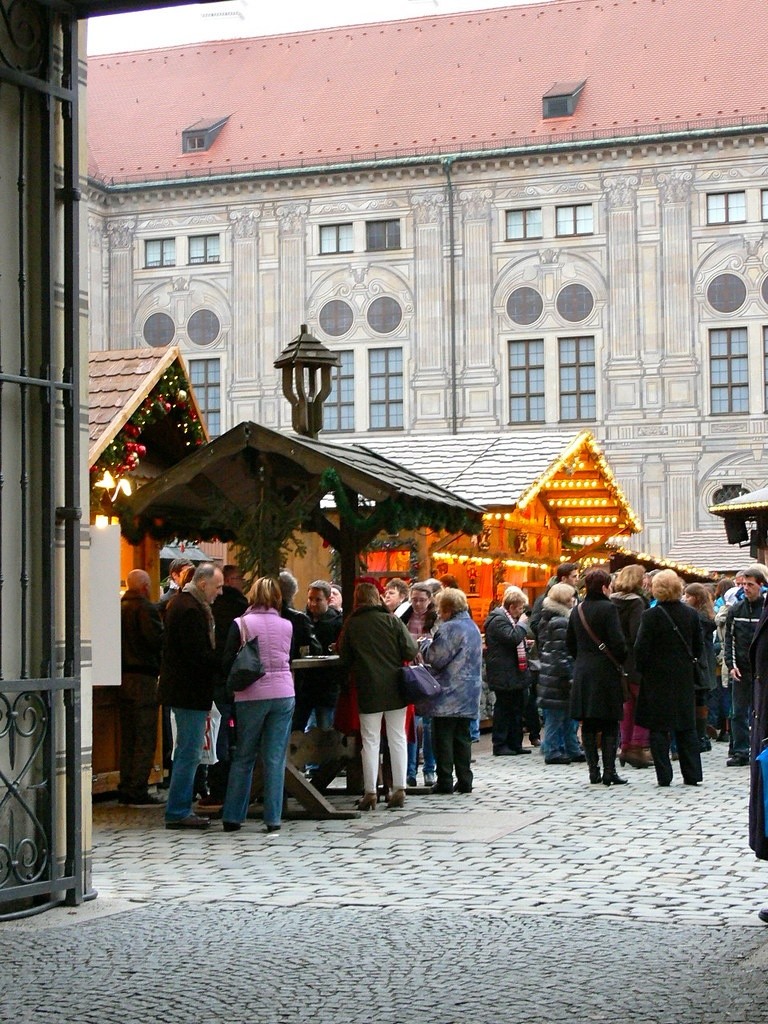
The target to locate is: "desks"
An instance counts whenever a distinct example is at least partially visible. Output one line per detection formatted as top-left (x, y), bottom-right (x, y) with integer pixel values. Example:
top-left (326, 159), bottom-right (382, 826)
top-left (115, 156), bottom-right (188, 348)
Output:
top-left (291, 655), bottom-right (341, 669)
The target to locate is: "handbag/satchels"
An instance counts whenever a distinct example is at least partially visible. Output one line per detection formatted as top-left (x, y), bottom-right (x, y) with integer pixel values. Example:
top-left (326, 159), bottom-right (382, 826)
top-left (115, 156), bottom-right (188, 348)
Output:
top-left (621, 676), bottom-right (631, 703)
top-left (692, 660), bottom-right (710, 693)
top-left (226, 616), bottom-right (266, 692)
top-left (402, 664), bottom-right (442, 705)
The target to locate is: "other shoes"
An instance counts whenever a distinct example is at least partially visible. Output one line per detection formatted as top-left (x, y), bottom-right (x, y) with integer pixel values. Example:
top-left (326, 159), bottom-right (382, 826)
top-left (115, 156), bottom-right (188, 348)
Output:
top-left (223, 821), bottom-right (240, 832)
top-left (424, 771), bottom-right (435, 786)
top-left (268, 824), bottom-right (281, 831)
top-left (572, 754), bottom-right (586, 762)
top-left (453, 783), bottom-right (472, 793)
top-left (545, 755), bottom-right (571, 764)
top-left (515, 747), bottom-right (531, 754)
top-left (408, 776), bottom-right (417, 787)
top-left (619, 725), bottom-right (749, 786)
top-left (531, 738), bottom-right (541, 745)
top-left (493, 745), bottom-right (516, 755)
top-left (432, 784), bottom-right (453, 794)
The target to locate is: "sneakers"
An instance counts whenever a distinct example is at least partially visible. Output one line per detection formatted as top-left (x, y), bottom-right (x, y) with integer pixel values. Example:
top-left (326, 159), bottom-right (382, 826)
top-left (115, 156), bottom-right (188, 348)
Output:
top-left (166, 814), bottom-right (210, 829)
top-left (117, 791), bottom-right (128, 807)
top-left (128, 794), bottom-right (166, 808)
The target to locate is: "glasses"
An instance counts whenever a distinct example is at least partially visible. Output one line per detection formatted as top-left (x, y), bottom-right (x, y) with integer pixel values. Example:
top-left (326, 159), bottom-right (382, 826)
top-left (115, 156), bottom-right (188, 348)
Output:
top-left (409, 597), bottom-right (429, 603)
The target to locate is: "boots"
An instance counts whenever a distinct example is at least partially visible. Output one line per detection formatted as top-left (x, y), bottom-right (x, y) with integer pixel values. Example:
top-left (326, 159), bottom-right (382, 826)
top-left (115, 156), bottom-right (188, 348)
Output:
top-left (600, 736), bottom-right (628, 785)
top-left (581, 733), bottom-right (602, 784)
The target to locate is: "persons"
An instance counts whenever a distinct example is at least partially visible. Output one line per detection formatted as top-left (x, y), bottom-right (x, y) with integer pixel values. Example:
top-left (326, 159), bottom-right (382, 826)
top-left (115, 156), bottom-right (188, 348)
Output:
top-left (277, 570), bottom-right (343, 778)
top-left (529, 563), bottom-right (768, 785)
top-left (221, 576), bottom-right (296, 833)
top-left (339, 583), bottom-right (418, 810)
top-left (209, 564), bottom-right (249, 764)
top-left (116, 568), bottom-right (167, 808)
top-left (153, 558), bottom-right (224, 827)
top-left (383, 575), bottom-right (483, 794)
top-left (483, 582), bottom-right (542, 756)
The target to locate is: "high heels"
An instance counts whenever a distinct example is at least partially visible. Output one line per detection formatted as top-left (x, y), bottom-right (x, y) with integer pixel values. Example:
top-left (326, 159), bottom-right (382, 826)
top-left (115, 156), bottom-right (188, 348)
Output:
top-left (386, 790), bottom-right (405, 809)
top-left (358, 792), bottom-right (377, 810)
